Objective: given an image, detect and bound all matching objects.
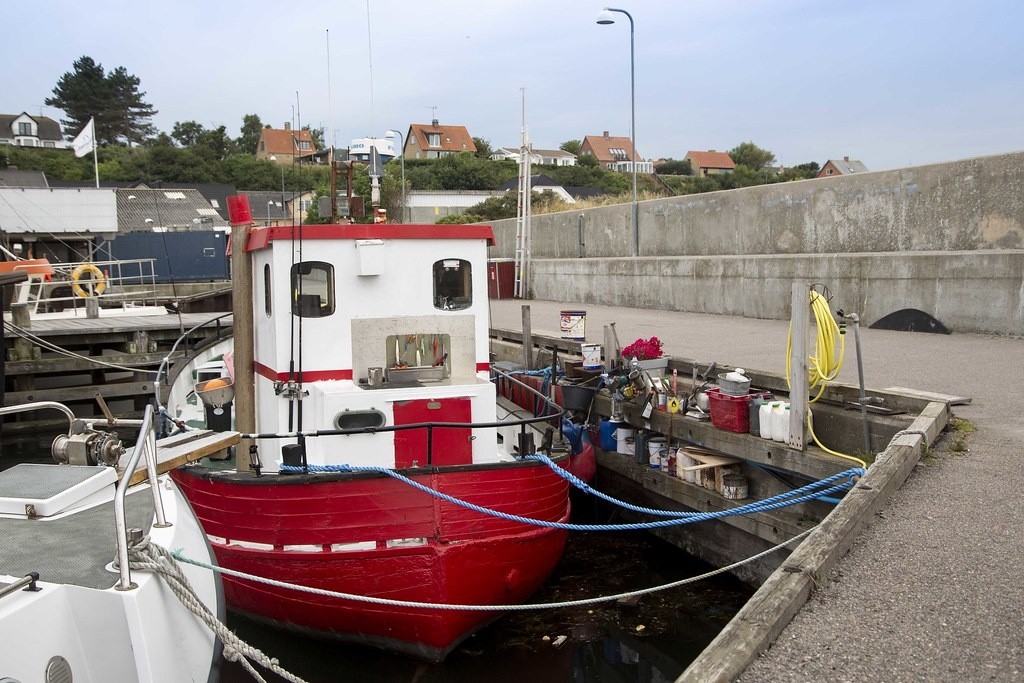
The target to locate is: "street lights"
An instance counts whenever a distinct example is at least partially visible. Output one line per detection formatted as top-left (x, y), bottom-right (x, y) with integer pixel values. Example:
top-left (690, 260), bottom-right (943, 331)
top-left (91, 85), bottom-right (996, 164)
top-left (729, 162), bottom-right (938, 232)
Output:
top-left (596, 6), bottom-right (639, 258)
top-left (385, 129), bottom-right (405, 224)
top-left (270, 156), bottom-right (285, 227)
top-left (268, 200), bottom-right (273, 227)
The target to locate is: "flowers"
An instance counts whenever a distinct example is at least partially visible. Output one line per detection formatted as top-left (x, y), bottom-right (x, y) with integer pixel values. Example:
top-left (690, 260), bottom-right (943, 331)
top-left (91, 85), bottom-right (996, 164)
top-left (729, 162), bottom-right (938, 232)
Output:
top-left (621, 336), bottom-right (665, 361)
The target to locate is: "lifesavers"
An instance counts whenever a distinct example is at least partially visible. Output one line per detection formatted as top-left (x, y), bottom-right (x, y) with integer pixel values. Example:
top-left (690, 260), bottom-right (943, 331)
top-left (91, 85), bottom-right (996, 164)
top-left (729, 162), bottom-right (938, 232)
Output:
top-left (71, 263), bottom-right (106, 298)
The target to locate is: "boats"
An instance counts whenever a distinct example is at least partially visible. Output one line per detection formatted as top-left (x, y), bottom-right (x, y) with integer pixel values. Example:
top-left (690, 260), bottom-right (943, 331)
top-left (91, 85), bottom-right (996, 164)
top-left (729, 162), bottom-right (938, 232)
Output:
top-left (0, 179), bottom-right (170, 321)
top-left (0, 393), bottom-right (226, 683)
top-left (156, 140), bottom-right (595, 665)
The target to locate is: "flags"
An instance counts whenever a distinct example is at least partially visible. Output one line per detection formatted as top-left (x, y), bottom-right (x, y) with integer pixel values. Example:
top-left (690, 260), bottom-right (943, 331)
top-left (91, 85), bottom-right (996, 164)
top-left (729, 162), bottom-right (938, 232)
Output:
top-left (73, 118), bottom-right (94, 157)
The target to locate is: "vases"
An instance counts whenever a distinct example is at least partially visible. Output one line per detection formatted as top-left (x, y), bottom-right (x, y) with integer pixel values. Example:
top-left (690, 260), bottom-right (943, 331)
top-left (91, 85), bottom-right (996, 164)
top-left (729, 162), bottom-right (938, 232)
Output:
top-left (624, 360), bottom-right (668, 379)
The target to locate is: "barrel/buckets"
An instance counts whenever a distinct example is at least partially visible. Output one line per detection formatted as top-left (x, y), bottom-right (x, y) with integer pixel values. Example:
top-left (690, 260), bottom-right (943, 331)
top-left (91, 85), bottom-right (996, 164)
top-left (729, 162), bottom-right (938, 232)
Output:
top-left (600, 420), bottom-right (748, 500)
top-left (581, 345), bottom-right (602, 370)
top-left (749, 398), bottom-right (813, 445)
top-left (559, 310), bottom-right (587, 341)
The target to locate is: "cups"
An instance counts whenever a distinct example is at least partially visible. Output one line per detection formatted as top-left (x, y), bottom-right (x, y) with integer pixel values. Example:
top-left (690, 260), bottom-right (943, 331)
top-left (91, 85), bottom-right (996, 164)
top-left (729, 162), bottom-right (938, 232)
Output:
top-left (367, 368), bottom-right (383, 386)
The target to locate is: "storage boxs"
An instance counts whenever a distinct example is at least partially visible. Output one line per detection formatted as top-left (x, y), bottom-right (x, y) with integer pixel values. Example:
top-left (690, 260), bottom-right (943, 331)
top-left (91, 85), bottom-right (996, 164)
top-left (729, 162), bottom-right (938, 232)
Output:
top-left (386, 366), bottom-right (445, 382)
top-left (704, 388), bottom-right (771, 431)
